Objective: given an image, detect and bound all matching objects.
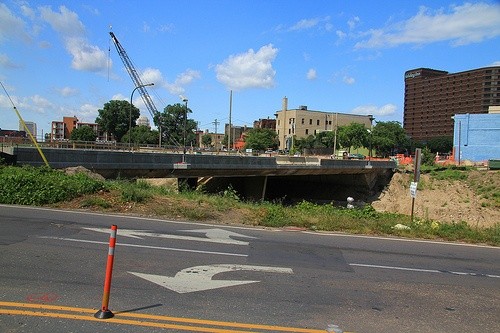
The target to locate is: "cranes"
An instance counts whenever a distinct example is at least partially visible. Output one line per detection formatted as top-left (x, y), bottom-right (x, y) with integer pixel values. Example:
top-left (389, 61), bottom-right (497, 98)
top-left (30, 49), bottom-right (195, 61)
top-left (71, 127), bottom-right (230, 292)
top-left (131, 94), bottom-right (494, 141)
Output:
top-left (107, 27), bottom-right (192, 171)
